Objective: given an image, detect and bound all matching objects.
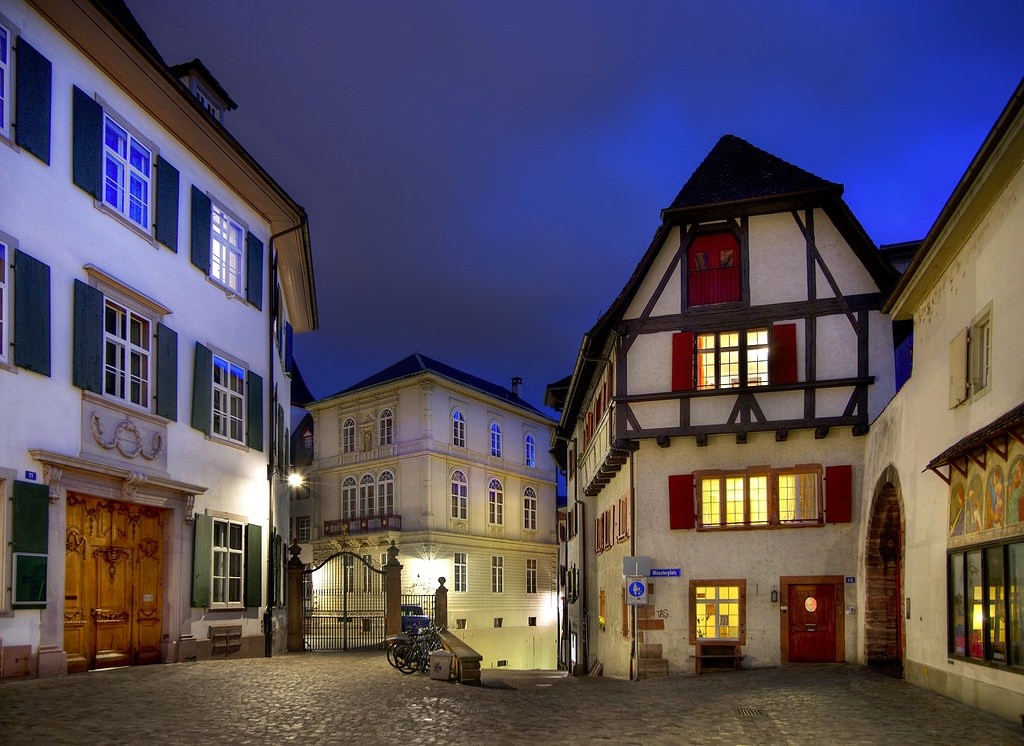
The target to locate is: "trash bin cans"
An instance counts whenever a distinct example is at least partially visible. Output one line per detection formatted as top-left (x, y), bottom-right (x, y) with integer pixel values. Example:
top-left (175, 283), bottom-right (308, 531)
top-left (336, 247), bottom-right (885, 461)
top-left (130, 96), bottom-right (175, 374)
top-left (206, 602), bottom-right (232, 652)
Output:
top-left (427, 648), bottom-right (456, 681)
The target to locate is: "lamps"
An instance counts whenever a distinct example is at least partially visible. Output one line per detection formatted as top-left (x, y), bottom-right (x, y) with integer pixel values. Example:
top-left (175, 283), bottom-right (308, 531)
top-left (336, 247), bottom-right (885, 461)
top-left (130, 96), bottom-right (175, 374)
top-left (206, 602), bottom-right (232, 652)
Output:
top-left (267, 464), bottom-right (304, 487)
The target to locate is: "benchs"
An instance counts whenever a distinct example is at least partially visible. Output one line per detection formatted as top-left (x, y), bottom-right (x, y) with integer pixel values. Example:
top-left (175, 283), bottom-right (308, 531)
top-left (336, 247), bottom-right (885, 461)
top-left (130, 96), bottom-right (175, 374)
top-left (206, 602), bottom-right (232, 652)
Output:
top-left (689, 655), bottom-right (745, 675)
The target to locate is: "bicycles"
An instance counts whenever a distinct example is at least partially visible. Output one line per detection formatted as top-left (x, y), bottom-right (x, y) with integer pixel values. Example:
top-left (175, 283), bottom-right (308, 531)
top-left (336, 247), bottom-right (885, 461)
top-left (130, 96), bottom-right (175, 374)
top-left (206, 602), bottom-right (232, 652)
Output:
top-left (387, 620), bottom-right (449, 674)
